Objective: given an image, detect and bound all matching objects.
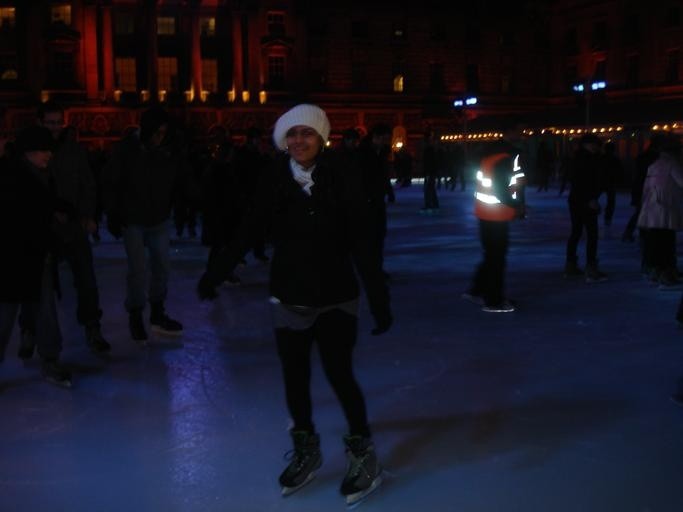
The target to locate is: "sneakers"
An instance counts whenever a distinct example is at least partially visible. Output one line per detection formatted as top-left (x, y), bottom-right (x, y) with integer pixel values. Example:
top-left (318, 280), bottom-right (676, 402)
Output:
top-left (461, 292), bottom-right (514, 313)
top-left (585, 272), bottom-right (609, 283)
top-left (648, 274), bottom-right (683, 290)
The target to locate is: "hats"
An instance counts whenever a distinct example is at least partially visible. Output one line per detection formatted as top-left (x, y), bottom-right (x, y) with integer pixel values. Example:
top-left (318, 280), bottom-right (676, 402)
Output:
top-left (272, 103), bottom-right (331, 151)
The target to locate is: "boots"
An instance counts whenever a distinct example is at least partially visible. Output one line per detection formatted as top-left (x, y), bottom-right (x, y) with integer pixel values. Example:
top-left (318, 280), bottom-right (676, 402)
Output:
top-left (279, 426), bottom-right (322, 487)
top-left (127, 305), bottom-right (148, 341)
top-left (150, 300), bottom-right (183, 331)
top-left (341, 424), bottom-right (382, 495)
